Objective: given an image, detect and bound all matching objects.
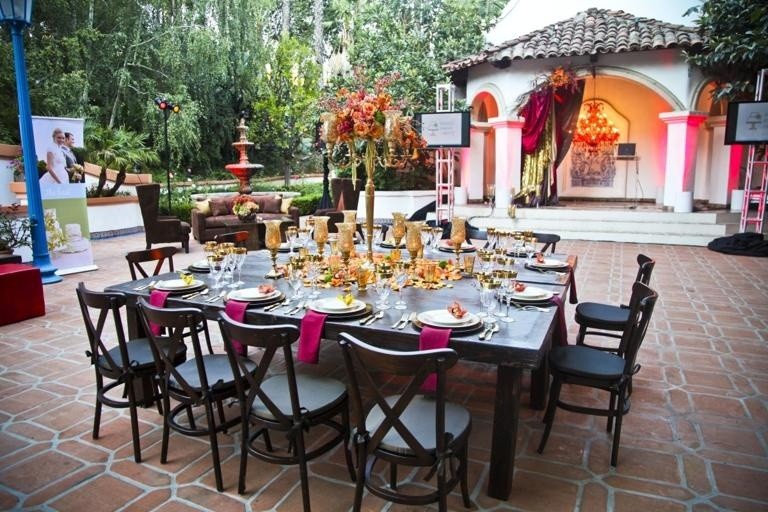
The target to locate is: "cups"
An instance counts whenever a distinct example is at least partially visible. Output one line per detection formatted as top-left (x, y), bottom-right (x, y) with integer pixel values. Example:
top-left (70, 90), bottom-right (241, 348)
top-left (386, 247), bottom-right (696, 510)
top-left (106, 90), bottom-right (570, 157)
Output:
top-left (464, 255), bottom-right (477, 277)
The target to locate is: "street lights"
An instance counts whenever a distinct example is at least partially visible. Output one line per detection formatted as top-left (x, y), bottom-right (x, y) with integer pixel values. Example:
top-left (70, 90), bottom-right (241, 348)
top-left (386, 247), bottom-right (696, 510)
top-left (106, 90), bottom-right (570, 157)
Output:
top-left (152, 93), bottom-right (178, 213)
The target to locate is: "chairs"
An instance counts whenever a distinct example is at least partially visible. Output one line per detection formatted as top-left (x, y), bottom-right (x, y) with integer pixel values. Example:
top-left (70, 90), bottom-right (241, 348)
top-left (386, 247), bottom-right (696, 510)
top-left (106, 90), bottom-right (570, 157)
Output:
top-left (136, 184), bottom-right (192, 254)
top-left (196, 194), bottom-right (282, 217)
top-left (310, 177), bottom-right (365, 242)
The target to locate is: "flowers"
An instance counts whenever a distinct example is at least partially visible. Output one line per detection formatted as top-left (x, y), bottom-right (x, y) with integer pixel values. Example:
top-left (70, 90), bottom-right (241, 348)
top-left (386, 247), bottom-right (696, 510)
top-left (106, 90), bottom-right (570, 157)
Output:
top-left (7, 146), bottom-right (48, 182)
top-left (303, 59), bottom-right (430, 191)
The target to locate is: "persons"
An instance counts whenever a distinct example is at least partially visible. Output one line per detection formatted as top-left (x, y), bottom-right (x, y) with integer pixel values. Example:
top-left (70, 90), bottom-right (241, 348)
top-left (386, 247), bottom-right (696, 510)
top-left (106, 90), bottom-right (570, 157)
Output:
top-left (60, 131), bottom-right (85, 184)
top-left (38, 128), bottom-right (70, 182)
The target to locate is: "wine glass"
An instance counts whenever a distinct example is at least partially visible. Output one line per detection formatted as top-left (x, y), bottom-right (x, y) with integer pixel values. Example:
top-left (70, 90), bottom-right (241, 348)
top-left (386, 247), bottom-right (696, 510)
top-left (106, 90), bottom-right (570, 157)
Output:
top-left (203, 241), bottom-right (247, 290)
top-left (285, 226), bottom-right (323, 300)
top-left (373, 268), bottom-right (408, 311)
top-left (358, 224), bottom-right (383, 253)
top-left (477, 226), bottom-right (539, 322)
top-left (421, 229), bottom-right (442, 258)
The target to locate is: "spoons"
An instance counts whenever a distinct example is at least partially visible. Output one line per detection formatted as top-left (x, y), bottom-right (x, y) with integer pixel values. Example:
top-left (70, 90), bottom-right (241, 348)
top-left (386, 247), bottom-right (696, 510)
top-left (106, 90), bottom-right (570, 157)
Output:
top-left (186, 287), bottom-right (210, 300)
top-left (486, 322), bottom-right (500, 341)
top-left (270, 297), bottom-right (290, 312)
top-left (365, 311), bottom-right (384, 328)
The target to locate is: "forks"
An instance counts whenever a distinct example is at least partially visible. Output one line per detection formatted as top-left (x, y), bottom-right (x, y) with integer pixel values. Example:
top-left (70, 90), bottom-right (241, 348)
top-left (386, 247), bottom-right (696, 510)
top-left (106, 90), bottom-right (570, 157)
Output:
top-left (390, 309), bottom-right (418, 330)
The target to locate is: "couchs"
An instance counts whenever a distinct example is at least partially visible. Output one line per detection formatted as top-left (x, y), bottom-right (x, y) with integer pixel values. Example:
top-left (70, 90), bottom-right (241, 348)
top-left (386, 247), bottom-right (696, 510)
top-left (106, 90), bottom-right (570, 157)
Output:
top-left (192, 198), bottom-right (300, 244)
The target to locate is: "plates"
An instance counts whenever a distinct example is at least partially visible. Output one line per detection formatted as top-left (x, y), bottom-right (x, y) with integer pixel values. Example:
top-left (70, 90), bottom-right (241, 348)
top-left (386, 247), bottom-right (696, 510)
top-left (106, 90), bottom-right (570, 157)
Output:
top-left (187, 260), bottom-right (236, 271)
top-left (504, 248), bottom-right (536, 259)
top-left (309, 296), bottom-right (366, 314)
top-left (153, 278), bottom-right (204, 291)
top-left (510, 284), bottom-right (552, 304)
top-left (417, 308), bottom-right (481, 328)
top-left (529, 256), bottom-right (570, 271)
top-left (436, 242), bottom-right (475, 254)
top-left (277, 242), bottom-right (303, 252)
top-left (227, 287), bottom-right (282, 301)
